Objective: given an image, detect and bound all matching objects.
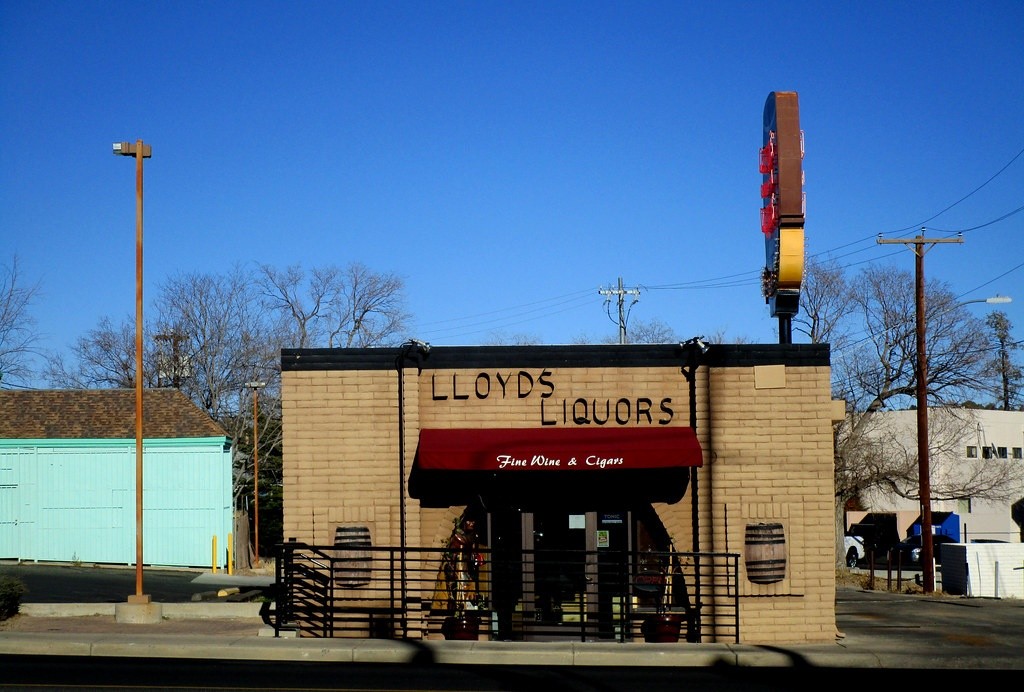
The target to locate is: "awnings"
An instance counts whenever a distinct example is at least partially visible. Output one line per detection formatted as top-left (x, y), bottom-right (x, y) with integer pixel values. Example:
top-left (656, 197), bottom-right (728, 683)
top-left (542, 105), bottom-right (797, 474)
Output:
top-left (418, 426), bottom-right (704, 470)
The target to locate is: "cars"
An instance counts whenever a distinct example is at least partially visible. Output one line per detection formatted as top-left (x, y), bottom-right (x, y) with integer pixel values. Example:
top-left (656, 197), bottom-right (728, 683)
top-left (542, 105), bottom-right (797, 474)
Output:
top-left (887, 534), bottom-right (960, 567)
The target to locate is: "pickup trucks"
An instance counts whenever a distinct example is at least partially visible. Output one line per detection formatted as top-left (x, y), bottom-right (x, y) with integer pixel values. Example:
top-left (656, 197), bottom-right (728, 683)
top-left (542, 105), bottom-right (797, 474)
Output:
top-left (843, 536), bottom-right (866, 567)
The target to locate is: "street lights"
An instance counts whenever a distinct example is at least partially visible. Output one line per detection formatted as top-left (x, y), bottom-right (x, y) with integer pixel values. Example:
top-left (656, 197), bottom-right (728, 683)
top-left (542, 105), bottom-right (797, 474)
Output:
top-left (395, 338), bottom-right (432, 627)
top-left (877, 227), bottom-right (1012, 594)
top-left (678, 335), bottom-right (711, 643)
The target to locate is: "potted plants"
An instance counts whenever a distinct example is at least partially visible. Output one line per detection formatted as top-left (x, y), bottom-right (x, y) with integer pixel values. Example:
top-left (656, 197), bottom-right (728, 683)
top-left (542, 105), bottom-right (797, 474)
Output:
top-left (441, 517), bottom-right (485, 640)
top-left (639, 533), bottom-right (685, 643)
